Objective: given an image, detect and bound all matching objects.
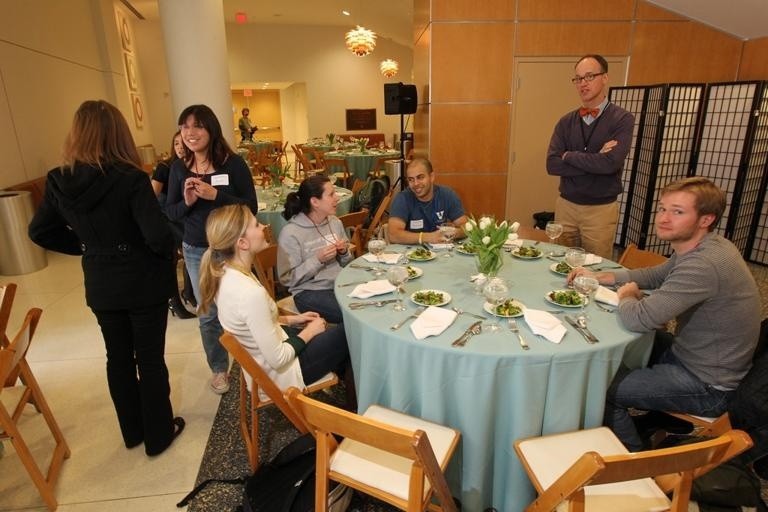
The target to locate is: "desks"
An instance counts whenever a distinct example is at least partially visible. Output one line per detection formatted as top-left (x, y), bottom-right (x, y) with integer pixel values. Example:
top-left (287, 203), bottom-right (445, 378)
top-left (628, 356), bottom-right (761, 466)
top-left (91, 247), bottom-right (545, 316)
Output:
top-left (334, 238), bottom-right (656, 511)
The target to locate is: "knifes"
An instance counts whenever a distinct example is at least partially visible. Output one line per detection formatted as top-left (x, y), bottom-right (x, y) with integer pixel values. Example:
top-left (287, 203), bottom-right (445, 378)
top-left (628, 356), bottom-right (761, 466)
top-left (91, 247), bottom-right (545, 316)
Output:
top-left (564, 315), bottom-right (593, 345)
top-left (350, 264), bottom-right (386, 272)
top-left (338, 280), bottom-right (367, 288)
top-left (452, 321), bottom-right (483, 346)
top-left (349, 299), bottom-right (401, 306)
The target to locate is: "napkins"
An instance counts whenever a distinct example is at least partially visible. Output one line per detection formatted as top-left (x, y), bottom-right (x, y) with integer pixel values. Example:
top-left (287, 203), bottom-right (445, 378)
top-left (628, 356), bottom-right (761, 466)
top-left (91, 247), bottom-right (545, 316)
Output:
top-left (523, 308), bottom-right (569, 344)
top-left (410, 304), bottom-right (458, 339)
top-left (583, 254), bottom-right (602, 266)
top-left (362, 252), bottom-right (403, 265)
top-left (594, 285), bottom-right (621, 307)
top-left (501, 238), bottom-right (522, 252)
top-left (348, 279), bottom-right (398, 299)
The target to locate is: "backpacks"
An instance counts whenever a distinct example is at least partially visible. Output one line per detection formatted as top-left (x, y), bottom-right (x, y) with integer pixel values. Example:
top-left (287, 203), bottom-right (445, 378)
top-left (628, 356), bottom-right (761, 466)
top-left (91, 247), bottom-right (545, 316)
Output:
top-left (351, 176), bottom-right (390, 228)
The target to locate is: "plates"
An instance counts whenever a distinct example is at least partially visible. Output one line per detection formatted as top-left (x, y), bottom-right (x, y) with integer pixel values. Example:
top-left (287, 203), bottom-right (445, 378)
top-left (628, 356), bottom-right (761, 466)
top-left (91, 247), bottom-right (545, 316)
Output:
top-left (512, 247), bottom-right (544, 259)
top-left (484, 299), bottom-right (526, 317)
top-left (410, 289), bottom-right (451, 307)
top-left (550, 263), bottom-right (586, 276)
top-left (407, 266), bottom-right (424, 280)
top-left (404, 249), bottom-right (436, 261)
top-left (456, 244), bottom-right (471, 255)
top-left (545, 290), bottom-right (590, 308)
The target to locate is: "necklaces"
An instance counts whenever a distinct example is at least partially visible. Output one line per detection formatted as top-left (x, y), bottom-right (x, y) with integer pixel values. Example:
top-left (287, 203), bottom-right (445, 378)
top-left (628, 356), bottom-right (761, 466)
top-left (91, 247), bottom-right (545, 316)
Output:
top-left (306, 214), bottom-right (338, 246)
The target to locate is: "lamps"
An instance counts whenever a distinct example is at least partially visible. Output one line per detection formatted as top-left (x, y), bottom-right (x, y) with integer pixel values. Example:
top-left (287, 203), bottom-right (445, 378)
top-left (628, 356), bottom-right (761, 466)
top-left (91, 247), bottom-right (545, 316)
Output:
top-left (344, 0), bottom-right (377, 57)
top-left (379, 36), bottom-right (400, 79)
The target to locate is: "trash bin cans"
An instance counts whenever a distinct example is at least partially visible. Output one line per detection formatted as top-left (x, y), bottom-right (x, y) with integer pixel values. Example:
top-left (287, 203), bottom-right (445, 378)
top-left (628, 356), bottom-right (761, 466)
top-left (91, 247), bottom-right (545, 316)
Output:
top-left (385, 159), bottom-right (402, 189)
top-left (136, 144), bottom-right (155, 166)
top-left (0, 190), bottom-right (47, 276)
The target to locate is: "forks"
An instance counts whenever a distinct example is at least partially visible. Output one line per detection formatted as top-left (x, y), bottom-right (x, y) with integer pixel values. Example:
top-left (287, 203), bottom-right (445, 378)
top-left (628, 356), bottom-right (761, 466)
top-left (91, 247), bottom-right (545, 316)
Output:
top-left (390, 306), bottom-right (426, 330)
top-left (591, 266), bottom-right (622, 272)
top-left (507, 318), bottom-right (529, 349)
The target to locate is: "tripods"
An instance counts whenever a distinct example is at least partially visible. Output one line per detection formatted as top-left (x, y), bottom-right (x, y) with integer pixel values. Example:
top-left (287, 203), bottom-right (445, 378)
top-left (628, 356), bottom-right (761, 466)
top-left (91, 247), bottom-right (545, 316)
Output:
top-left (382, 115), bottom-right (407, 224)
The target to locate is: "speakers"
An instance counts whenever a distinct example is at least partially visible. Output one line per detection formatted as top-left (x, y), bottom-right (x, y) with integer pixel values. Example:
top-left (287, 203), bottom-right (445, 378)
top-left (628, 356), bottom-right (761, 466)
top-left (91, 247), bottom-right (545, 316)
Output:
top-left (384, 83), bottom-right (417, 114)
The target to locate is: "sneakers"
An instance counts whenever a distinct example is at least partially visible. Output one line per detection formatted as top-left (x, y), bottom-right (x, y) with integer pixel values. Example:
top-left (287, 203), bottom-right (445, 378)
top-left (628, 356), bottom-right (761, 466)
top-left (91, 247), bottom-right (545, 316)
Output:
top-left (212, 372), bottom-right (229, 394)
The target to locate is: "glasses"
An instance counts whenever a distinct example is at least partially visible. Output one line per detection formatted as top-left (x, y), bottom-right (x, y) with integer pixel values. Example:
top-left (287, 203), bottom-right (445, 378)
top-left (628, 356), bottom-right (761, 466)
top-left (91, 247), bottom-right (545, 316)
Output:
top-left (572, 72), bottom-right (603, 83)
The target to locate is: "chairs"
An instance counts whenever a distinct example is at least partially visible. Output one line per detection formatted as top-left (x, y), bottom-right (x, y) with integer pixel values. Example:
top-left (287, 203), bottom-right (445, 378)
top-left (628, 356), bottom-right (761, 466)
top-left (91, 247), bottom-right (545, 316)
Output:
top-left (512, 425), bottom-right (752, 511)
top-left (1, 282), bottom-right (43, 414)
top-left (667, 411), bottom-right (732, 438)
top-left (618, 245), bottom-right (671, 272)
top-left (0, 307), bottom-right (71, 512)
top-left (219, 328), bottom-right (339, 474)
top-left (217, 140), bottom-right (408, 332)
top-left (286, 386), bottom-right (459, 512)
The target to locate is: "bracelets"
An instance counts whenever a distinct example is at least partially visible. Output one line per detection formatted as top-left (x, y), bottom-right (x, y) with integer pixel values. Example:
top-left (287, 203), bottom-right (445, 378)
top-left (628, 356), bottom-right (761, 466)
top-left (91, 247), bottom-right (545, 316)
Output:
top-left (419, 232), bottom-right (423, 245)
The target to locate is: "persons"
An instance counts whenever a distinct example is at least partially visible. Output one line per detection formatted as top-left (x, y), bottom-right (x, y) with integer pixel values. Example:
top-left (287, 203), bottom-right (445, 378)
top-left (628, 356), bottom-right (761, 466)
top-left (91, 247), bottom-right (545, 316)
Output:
top-left (164, 105), bottom-right (258, 394)
top-left (388, 157), bottom-right (469, 245)
top-left (151, 131), bottom-right (197, 319)
top-left (568, 177), bottom-right (762, 453)
top-left (546, 55), bottom-right (635, 260)
top-left (277, 176), bottom-right (356, 324)
top-left (28, 100), bottom-right (186, 456)
top-left (239, 108), bottom-right (258, 141)
top-left (197, 205), bottom-right (358, 414)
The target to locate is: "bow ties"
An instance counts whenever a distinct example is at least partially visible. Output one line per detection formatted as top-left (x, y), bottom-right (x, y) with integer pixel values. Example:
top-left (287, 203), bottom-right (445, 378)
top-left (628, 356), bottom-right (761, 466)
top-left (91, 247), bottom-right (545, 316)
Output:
top-left (580, 108), bottom-right (599, 118)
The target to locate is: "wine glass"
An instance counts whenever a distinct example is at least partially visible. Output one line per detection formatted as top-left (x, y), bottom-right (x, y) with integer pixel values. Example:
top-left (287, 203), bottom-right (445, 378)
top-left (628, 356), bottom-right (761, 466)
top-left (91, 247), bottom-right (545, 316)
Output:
top-left (485, 270), bottom-right (508, 332)
top-left (386, 266), bottom-right (409, 312)
top-left (545, 220), bottom-right (563, 256)
top-left (369, 236), bottom-right (386, 275)
top-left (572, 272), bottom-right (599, 322)
top-left (439, 223), bottom-right (456, 258)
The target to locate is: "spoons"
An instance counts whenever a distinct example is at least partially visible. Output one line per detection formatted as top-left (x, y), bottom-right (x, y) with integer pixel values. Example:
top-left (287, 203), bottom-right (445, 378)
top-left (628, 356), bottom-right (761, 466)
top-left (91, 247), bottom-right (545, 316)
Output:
top-left (351, 301), bottom-right (386, 309)
top-left (576, 317), bottom-right (599, 341)
top-left (449, 305), bottom-right (486, 319)
top-left (457, 325), bottom-right (481, 347)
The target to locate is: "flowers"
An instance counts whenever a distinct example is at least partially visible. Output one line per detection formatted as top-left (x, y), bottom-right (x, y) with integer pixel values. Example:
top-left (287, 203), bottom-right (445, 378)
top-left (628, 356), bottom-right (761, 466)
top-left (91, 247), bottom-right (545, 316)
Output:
top-left (461, 213), bottom-right (521, 276)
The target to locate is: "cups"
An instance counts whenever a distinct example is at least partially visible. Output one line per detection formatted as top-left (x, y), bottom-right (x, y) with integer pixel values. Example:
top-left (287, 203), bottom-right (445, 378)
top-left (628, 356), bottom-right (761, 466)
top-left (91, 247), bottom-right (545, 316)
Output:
top-left (255, 171), bottom-right (337, 210)
top-left (565, 247), bottom-right (586, 268)
top-left (306, 136), bottom-right (391, 156)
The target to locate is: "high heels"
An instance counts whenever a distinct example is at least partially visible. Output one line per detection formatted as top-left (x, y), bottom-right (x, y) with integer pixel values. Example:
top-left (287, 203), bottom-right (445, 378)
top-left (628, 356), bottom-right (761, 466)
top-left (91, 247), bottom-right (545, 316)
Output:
top-left (182, 291), bottom-right (196, 306)
top-left (169, 296), bottom-right (195, 318)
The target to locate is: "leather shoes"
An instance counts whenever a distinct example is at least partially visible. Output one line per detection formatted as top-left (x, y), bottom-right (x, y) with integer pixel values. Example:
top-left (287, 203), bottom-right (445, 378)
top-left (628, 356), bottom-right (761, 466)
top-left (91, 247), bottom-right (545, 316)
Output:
top-left (173, 417), bottom-right (184, 436)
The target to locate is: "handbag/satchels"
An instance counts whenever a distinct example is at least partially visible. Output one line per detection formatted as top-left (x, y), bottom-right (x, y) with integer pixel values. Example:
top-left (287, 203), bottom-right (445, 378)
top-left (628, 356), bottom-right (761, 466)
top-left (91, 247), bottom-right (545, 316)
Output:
top-left (243, 432), bottom-right (355, 512)
top-left (661, 435), bottom-right (768, 511)
